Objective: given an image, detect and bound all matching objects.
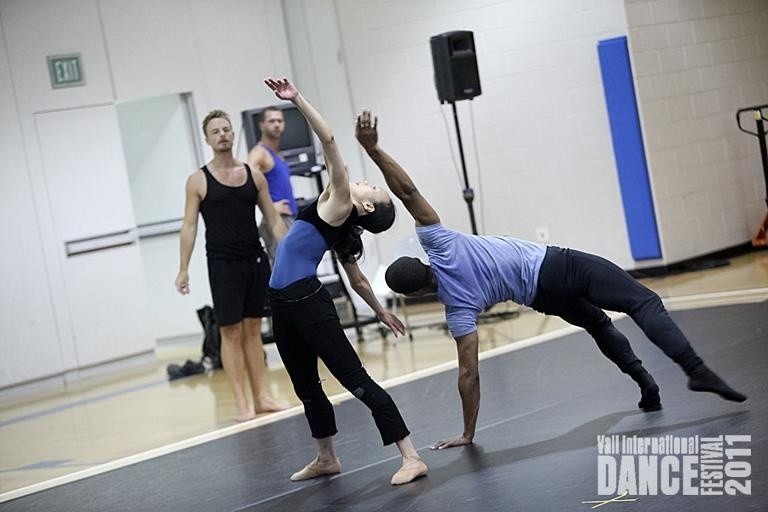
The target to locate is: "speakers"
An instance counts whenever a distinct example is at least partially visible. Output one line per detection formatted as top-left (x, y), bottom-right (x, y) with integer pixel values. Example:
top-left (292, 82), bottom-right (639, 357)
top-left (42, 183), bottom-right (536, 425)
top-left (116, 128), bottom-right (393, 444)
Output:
top-left (429, 30), bottom-right (482, 103)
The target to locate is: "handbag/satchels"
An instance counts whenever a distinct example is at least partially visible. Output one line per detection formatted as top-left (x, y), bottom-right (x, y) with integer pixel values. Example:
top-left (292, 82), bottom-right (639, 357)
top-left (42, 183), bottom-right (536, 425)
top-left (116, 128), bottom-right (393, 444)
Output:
top-left (198, 306), bottom-right (223, 368)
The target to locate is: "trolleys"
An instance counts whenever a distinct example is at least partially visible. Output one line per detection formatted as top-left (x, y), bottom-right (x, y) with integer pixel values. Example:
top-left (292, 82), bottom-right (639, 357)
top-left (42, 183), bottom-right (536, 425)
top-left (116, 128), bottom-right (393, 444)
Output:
top-left (736, 102), bottom-right (767, 247)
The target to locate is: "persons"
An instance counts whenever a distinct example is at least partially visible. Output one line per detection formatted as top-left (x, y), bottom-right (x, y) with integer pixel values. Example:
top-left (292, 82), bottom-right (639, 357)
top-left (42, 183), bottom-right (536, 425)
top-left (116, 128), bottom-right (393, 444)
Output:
top-left (354, 110), bottom-right (748, 452)
top-left (263, 76), bottom-right (429, 486)
top-left (244, 104), bottom-right (299, 262)
top-left (172, 110), bottom-right (291, 422)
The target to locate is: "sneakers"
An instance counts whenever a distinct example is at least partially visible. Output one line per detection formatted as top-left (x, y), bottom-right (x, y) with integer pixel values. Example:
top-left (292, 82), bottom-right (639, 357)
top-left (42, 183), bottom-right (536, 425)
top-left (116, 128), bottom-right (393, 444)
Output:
top-left (167, 361), bottom-right (204, 378)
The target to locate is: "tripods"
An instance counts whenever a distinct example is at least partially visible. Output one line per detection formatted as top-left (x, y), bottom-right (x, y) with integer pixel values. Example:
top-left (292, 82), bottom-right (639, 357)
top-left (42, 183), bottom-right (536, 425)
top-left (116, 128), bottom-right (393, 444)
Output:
top-left (443, 103), bottom-right (519, 332)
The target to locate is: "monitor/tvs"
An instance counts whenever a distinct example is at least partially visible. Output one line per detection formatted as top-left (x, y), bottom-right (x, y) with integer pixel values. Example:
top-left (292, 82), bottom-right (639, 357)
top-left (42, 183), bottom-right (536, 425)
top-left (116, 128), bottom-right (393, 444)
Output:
top-left (241, 99), bottom-right (317, 174)
